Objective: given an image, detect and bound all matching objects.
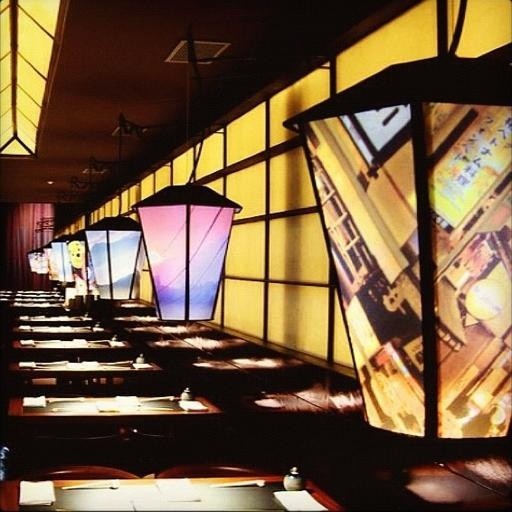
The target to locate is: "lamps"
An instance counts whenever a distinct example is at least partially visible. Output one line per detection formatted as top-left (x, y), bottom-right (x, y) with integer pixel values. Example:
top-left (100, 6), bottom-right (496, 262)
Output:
top-left (130, 49), bottom-right (243, 322)
top-left (80, 119), bottom-right (143, 301)
top-left (281, 0), bottom-right (512, 459)
top-left (27, 241), bottom-right (75, 283)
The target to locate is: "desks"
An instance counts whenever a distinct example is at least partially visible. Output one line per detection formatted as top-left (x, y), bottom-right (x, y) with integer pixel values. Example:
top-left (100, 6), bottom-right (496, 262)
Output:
top-left (0, 290), bottom-right (512, 512)
